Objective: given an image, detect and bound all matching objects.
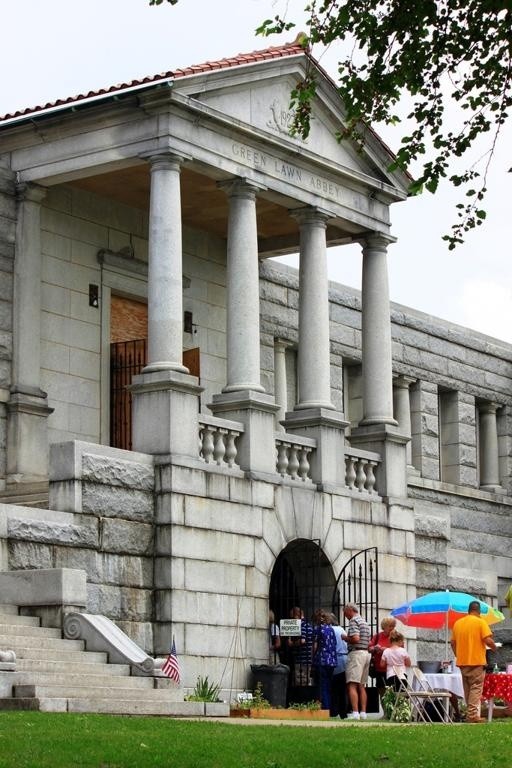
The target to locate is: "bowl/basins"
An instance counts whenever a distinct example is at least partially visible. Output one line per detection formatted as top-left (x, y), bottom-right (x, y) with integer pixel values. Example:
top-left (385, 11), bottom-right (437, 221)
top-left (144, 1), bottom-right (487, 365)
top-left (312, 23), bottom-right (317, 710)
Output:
top-left (417, 660), bottom-right (442, 673)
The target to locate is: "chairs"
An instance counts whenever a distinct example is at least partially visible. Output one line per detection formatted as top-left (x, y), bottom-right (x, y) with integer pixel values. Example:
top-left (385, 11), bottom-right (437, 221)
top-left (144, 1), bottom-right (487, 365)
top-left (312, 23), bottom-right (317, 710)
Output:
top-left (387, 664), bottom-right (455, 725)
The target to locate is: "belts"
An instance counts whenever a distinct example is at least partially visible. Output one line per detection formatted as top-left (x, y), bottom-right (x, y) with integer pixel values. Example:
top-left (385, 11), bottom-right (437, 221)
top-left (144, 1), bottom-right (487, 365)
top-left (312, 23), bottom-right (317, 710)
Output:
top-left (349, 648), bottom-right (367, 652)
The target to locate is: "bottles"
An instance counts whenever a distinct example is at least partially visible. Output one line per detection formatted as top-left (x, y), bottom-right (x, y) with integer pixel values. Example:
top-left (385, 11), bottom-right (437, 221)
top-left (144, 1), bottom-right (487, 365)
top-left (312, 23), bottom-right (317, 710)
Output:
top-left (443, 661), bottom-right (452, 673)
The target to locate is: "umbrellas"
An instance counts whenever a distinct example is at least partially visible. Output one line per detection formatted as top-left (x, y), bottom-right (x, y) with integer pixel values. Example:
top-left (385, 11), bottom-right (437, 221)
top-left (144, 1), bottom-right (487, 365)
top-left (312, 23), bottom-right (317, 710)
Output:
top-left (390, 588), bottom-right (505, 720)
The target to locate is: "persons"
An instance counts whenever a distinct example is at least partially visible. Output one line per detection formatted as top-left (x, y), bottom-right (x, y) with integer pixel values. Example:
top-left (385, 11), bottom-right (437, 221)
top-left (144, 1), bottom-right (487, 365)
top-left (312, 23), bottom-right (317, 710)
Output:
top-left (268, 605), bottom-right (412, 720)
top-left (451, 602), bottom-right (496, 720)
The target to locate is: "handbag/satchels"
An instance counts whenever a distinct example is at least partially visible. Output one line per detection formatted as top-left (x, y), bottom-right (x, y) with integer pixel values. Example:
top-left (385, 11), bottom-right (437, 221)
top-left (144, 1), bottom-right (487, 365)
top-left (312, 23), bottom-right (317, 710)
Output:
top-left (369, 634), bottom-right (377, 678)
top-left (310, 664), bottom-right (318, 678)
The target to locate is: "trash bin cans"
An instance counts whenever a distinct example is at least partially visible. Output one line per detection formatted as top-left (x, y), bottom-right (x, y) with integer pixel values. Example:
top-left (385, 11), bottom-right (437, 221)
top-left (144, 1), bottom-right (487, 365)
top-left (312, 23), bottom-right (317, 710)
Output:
top-left (250, 663), bottom-right (291, 709)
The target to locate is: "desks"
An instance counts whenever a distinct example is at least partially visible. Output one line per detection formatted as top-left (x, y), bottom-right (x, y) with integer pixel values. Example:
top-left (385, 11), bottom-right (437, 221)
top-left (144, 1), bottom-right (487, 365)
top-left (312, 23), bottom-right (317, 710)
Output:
top-left (411, 672), bottom-right (512, 724)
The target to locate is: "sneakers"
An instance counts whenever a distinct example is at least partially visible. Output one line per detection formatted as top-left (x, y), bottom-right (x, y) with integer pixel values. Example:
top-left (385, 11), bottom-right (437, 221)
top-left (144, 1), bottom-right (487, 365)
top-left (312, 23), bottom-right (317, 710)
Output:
top-left (344, 714), bottom-right (361, 721)
top-left (467, 717), bottom-right (485, 723)
top-left (347, 713), bottom-right (367, 720)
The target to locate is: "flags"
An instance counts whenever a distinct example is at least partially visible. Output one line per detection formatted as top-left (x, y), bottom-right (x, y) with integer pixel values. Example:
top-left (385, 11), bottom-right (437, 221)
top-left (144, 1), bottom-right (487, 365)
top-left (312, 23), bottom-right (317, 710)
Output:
top-left (162, 638), bottom-right (180, 685)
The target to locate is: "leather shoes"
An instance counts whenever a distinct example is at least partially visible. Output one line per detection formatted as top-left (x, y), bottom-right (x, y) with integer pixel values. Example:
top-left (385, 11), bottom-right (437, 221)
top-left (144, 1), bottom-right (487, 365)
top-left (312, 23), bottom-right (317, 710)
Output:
top-left (379, 716), bottom-right (389, 720)
